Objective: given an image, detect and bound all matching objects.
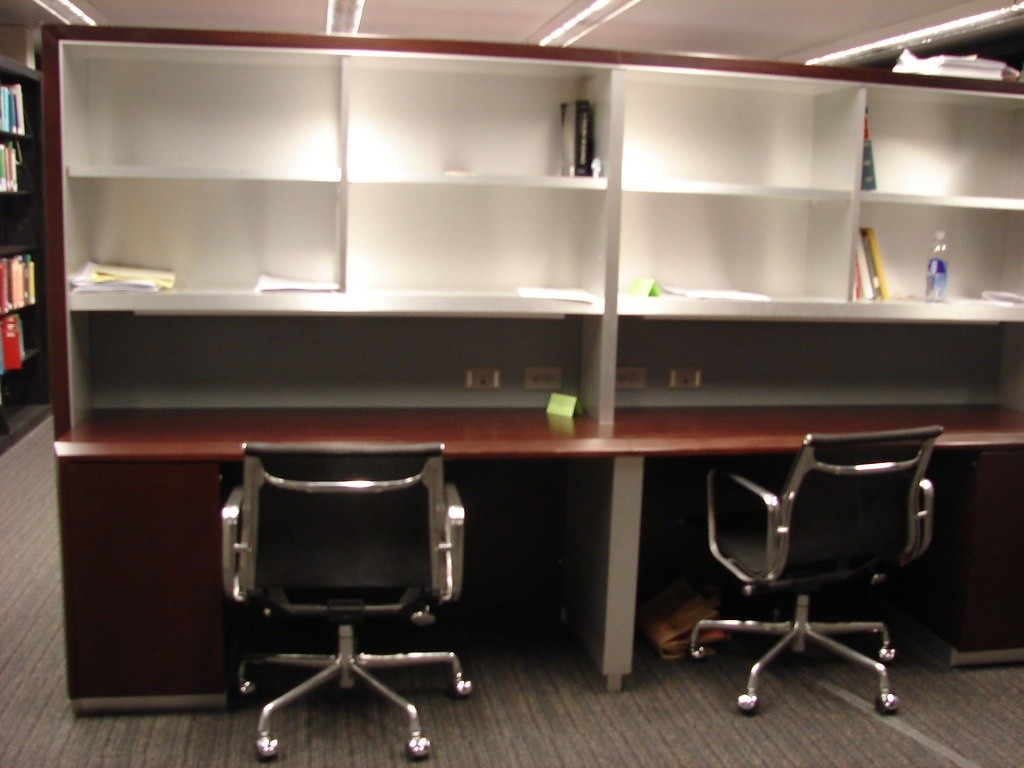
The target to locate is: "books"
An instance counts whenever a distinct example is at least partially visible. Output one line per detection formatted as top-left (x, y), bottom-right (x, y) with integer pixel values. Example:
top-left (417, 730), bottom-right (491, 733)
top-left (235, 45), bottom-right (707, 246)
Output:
top-left (0, 84), bottom-right (29, 192)
top-left (0, 256), bottom-right (37, 371)
top-left (857, 228), bottom-right (890, 300)
top-left (559, 100), bottom-right (592, 177)
top-left (861, 107), bottom-right (877, 191)
top-left (71, 260), bottom-right (174, 294)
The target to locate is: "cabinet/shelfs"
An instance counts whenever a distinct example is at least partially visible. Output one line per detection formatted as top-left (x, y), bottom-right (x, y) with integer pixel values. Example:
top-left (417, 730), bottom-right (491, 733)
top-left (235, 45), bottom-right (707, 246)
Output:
top-left (37, 20), bottom-right (1024, 701)
top-left (0, 51), bottom-right (53, 459)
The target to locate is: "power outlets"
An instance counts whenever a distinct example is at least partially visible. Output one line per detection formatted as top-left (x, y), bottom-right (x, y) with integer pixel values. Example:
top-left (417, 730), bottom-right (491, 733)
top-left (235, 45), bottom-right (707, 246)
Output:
top-left (616, 368), bottom-right (647, 388)
top-left (669, 368), bottom-right (701, 389)
top-left (524, 365), bottom-right (564, 390)
top-left (464, 369), bottom-right (501, 390)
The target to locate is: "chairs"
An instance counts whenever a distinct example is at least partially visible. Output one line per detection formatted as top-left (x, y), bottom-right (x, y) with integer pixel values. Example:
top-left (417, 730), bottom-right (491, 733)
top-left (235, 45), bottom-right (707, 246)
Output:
top-left (692, 424), bottom-right (947, 716)
top-left (218, 438), bottom-right (474, 761)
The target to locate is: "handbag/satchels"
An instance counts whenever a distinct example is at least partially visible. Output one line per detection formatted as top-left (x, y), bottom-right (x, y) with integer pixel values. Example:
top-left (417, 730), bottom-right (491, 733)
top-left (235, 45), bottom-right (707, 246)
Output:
top-left (636, 579), bottom-right (730, 660)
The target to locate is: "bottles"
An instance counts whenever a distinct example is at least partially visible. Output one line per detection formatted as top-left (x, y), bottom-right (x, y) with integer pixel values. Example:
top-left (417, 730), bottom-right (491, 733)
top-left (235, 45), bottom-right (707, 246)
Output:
top-left (924, 229), bottom-right (950, 302)
